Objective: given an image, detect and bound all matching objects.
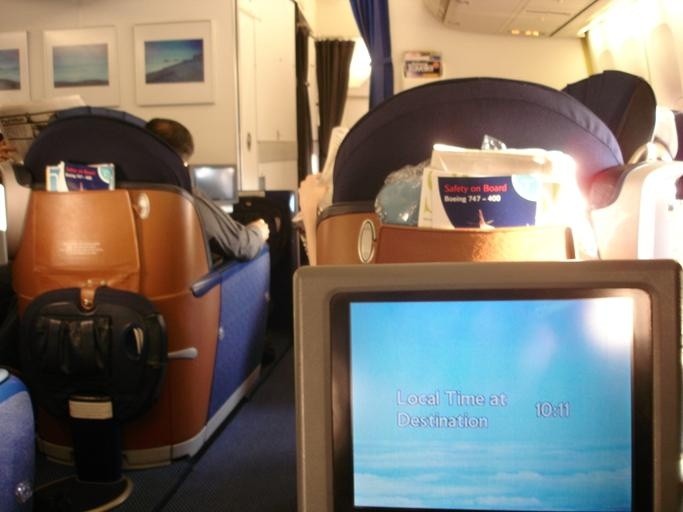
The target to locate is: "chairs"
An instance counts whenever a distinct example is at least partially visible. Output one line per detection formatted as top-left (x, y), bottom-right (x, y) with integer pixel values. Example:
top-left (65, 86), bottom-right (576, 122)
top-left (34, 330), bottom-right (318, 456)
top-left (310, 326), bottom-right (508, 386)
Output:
top-left (315, 75), bottom-right (627, 268)
top-left (8, 105), bottom-right (273, 472)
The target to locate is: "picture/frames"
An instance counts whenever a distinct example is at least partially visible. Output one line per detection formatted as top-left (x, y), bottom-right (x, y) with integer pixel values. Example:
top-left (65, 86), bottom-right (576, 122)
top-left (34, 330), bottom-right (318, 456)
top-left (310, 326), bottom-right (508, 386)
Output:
top-left (0, 18), bottom-right (215, 110)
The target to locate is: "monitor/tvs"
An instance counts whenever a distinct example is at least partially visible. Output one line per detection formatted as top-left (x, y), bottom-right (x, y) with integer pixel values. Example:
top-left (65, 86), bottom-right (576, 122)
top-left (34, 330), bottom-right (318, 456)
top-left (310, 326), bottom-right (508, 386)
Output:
top-left (188, 163), bottom-right (238, 205)
top-left (292, 258), bottom-right (683, 512)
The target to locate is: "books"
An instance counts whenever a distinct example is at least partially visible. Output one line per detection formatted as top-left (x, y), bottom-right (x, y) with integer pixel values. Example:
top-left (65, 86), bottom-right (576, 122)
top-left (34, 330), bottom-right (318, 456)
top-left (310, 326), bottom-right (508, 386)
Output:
top-left (43, 165), bottom-right (115, 193)
top-left (412, 138), bottom-right (575, 232)
top-left (319, 128), bottom-right (352, 211)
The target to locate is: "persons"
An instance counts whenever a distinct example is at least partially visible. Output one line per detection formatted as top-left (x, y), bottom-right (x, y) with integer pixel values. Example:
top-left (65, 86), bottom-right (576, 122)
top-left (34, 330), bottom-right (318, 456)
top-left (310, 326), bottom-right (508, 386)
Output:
top-left (148, 117), bottom-right (271, 263)
top-left (296, 173), bottom-right (325, 266)
